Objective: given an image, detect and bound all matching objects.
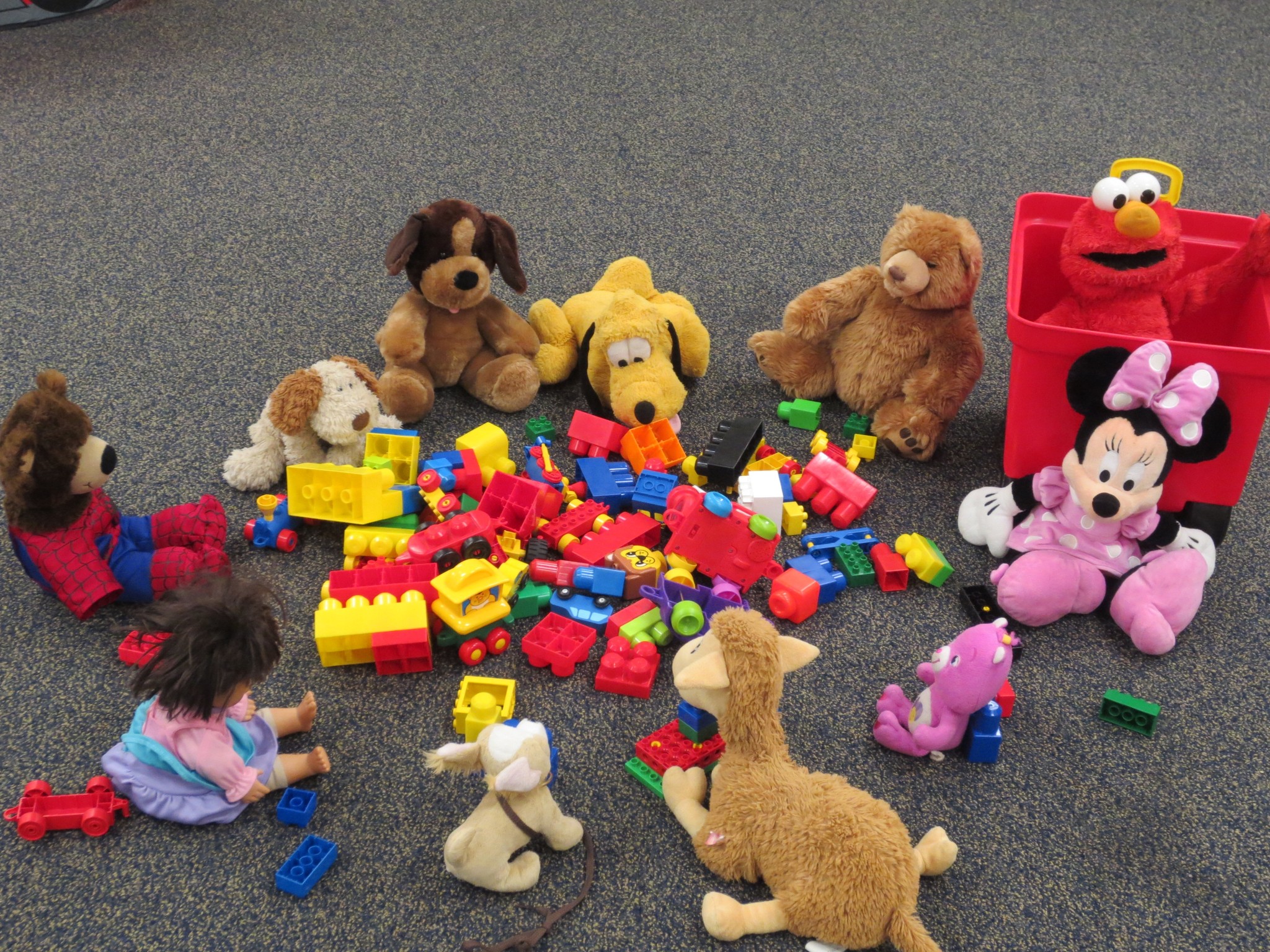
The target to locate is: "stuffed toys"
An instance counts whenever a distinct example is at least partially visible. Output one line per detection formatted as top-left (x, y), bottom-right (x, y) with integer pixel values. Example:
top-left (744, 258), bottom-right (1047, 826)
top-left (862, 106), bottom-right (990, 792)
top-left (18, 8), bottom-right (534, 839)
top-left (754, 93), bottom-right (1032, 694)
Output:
top-left (376, 198), bottom-right (540, 424)
top-left (747, 203), bottom-right (985, 463)
top-left (872, 617), bottom-right (1020, 763)
top-left (422, 718), bottom-right (584, 893)
top-left (1032, 172), bottom-right (1270, 340)
top-left (662, 605), bottom-right (958, 952)
top-left (527, 256), bottom-right (710, 434)
top-left (0, 369), bottom-right (233, 622)
top-left (957, 339), bottom-right (1232, 657)
top-left (221, 354), bottom-right (403, 492)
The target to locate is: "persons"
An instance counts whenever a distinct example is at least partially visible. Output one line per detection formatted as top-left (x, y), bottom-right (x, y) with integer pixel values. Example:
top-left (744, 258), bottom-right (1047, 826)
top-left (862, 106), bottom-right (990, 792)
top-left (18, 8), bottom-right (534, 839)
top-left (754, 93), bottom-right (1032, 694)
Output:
top-left (101, 560), bottom-right (331, 827)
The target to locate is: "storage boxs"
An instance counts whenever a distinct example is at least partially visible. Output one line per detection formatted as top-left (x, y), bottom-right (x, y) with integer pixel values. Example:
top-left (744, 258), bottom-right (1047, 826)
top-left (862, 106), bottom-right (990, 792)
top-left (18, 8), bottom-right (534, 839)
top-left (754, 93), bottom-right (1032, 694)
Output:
top-left (996, 186), bottom-right (1269, 548)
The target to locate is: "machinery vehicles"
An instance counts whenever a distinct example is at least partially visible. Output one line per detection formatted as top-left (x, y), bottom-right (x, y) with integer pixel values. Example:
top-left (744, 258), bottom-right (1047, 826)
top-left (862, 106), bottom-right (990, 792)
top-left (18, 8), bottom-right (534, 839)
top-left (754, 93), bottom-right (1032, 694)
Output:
top-left (428, 556), bottom-right (532, 668)
